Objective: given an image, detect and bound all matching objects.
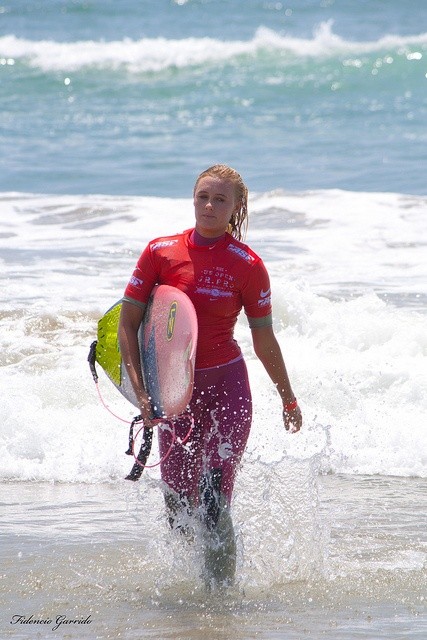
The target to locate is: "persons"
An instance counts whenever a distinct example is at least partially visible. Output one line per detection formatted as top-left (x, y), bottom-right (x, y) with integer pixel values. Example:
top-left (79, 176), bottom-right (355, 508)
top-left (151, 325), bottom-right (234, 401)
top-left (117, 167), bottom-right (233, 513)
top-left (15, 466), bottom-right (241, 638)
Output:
top-left (118, 164), bottom-right (302, 591)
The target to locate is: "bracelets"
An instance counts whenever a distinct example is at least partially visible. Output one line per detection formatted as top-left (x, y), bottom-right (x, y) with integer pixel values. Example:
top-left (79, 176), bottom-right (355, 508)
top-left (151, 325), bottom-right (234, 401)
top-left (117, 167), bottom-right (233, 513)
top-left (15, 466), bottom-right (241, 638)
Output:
top-left (282, 398), bottom-right (297, 410)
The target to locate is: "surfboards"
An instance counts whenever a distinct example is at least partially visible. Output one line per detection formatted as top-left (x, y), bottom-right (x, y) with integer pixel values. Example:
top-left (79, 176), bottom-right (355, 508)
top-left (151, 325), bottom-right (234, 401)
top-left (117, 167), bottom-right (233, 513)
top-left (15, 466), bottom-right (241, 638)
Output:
top-left (94, 285), bottom-right (199, 418)
